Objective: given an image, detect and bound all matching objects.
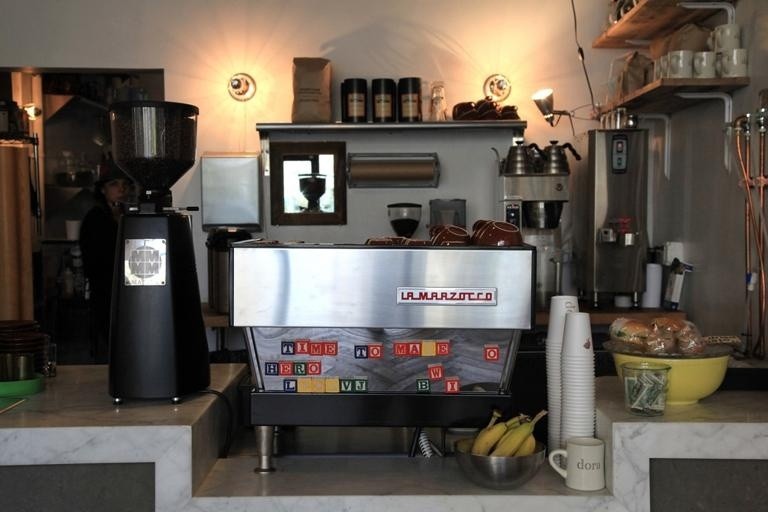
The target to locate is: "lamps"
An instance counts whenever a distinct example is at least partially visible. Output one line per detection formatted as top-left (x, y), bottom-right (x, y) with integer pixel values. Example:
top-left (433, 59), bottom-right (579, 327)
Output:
top-left (533, 88), bottom-right (569, 128)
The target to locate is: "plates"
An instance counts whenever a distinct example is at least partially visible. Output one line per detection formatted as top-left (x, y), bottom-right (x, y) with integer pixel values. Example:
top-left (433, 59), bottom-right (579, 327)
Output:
top-left (0, 319), bottom-right (46, 373)
top-left (0, 371), bottom-right (45, 399)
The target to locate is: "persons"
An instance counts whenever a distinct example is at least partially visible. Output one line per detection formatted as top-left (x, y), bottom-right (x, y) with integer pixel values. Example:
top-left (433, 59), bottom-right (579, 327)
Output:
top-left (80, 168), bottom-right (135, 363)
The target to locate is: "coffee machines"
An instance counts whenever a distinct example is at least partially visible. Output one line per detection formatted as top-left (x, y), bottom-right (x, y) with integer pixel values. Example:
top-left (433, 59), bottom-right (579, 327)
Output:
top-left (103, 98), bottom-right (212, 404)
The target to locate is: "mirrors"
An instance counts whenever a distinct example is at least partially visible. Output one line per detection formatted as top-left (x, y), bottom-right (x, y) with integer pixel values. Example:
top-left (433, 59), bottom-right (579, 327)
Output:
top-left (270, 141), bottom-right (347, 225)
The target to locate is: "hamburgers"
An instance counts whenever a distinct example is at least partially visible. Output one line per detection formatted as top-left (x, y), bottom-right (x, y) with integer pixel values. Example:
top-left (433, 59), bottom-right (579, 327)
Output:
top-left (606, 314), bottom-right (703, 359)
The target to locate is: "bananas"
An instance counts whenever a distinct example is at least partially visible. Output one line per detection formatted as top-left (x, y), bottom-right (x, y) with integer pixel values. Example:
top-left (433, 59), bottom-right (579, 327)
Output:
top-left (470, 408), bottom-right (550, 459)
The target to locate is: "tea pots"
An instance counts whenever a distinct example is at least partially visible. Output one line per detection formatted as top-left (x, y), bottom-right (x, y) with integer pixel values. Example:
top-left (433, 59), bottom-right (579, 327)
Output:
top-left (533, 139), bottom-right (582, 175)
top-left (488, 139), bottom-right (548, 176)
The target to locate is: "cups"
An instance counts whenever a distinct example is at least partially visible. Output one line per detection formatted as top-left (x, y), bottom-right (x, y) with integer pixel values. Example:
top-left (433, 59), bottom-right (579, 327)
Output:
top-left (542, 296), bottom-right (607, 491)
top-left (619, 359), bottom-right (671, 418)
top-left (649, 22), bottom-right (746, 83)
top-left (599, 106), bottom-right (636, 129)
top-left (450, 96), bottom-right (519, 120)
top-left (63, 219), bottom-right (81, 241)
top-left (613, 295), bottom-right (632, 309)
top-left (41, 342), bottom-right (58, 377)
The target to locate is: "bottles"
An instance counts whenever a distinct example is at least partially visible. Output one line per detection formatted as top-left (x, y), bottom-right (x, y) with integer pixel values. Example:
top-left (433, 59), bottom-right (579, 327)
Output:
top-left (426, 79), bottom-right (446, 123)
top-left (61, 267), bottom-right (73, 292)
top-left (60, 148), bottom-right (91, 175)
top-left (339, 77), bottom-right (422, 125)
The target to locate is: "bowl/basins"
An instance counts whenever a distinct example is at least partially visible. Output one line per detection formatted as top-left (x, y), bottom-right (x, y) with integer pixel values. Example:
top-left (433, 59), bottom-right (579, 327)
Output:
top-left (520, 198), bottom-right (569, 230)
top-left (610, 349), bottom-right (731, 406)
top-left (365, 219), bottom-right (520, 249)
top-left (0, 353), bottom-right (34, 381)
top-left (451, 436), bottom-right (548, 491)
top-left (53, 170), bottom-right (92, 187)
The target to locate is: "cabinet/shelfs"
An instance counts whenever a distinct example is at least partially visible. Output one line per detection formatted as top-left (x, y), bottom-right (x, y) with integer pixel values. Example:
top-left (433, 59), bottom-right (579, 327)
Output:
top-left (592, 1), bottom-right (749, 115)
